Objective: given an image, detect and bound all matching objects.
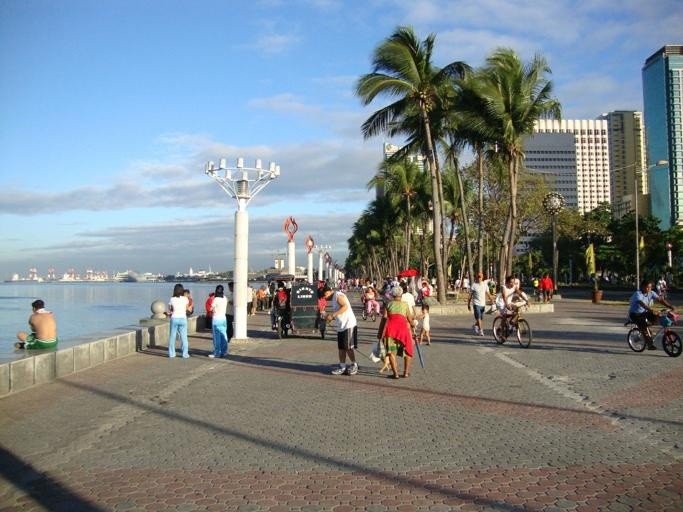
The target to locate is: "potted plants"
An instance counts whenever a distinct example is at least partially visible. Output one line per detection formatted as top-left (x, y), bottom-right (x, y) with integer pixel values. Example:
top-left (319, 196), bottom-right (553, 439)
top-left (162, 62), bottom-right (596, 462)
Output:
top-left (589, 273), bottom-right (604, 304)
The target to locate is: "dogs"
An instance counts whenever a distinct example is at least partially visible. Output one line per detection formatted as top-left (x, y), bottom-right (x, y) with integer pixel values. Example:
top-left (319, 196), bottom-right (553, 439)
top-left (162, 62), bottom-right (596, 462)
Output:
top-left (369, 349), bottom-right (399, 373)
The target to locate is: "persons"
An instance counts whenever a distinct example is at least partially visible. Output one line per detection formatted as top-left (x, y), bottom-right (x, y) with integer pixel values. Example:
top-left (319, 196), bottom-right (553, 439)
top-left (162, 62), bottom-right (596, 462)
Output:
top-left (337, 277), bottom-right (381, 316)
top-left (317, 286), bottom-right (361, 376)
top-left (225, 282), bottom-right (234, 340)
top-left (448, 274), bottom-right (469, 292)
top-left (207, 285), bottom-right (228, 358)
top-left (163, 289), bottom-right (194, 317)
top-left (532, 273), bottom-right (554, 303)
top-left (271, 278), bottom-right (293, 336)
top-left (247, 279), bottom-right (275, 316)
top-left (468, 274), bottom-right (529, 343)
top-left (204, 293), bottom-right (214, 329)
top-left (628, 282), bottom-right (676, 351)
top-left (382, 275), bottom-right (437, 345)
top-left (168, 284), bottom-right (190, 359)
top-left (376, 286), bottom-right (417, 378)
top-left (317, 279), bottom-right (330, 311)
top-left (655, 275), bottom-right (668, 296)
top-left (13, 300), bottom-right (57, 350)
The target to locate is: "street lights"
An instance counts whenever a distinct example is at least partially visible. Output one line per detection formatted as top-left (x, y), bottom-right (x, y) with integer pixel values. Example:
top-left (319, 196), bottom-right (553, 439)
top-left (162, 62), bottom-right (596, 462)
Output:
top-left (204, 158), bottom-right (280, 342)
top-left (664, 239), bottom-right (674, 271)
top-left (633, 159), bottom-right (668, 292)
top-left (418, 234), bottom-right (425, 280)
top-left (542, 191), bottom-right (564, 300)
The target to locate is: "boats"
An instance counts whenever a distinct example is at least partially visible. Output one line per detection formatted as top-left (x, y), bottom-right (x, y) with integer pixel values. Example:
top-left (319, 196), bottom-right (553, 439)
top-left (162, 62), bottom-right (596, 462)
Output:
top-left (164, 274), bottom-right (200, 282)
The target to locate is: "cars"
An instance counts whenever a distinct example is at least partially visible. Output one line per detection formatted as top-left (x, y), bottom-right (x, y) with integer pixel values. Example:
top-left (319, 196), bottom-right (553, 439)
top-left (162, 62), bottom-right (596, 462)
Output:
top-left (598, 275), bottom-right (623, 284)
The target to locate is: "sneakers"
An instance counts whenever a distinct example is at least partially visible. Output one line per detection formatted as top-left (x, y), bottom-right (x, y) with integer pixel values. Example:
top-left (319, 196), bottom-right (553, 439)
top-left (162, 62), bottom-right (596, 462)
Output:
top-left (478, 329), bottom-right (483, 336)
top-left (330, 367), bottom-right (347, 375)
top-left (347, 365), bottom-right (359, 376)
top-left (208, 354), bottom-right (221, 358)
top-left (473, 324), bottom-right (479, 334)
top-left (648, 346), bottom-right (657, 350)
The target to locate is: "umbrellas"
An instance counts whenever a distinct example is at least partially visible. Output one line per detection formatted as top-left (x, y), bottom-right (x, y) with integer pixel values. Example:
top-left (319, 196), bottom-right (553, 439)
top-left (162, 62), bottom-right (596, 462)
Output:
top-left (396, 269), bottom-right (421, 278)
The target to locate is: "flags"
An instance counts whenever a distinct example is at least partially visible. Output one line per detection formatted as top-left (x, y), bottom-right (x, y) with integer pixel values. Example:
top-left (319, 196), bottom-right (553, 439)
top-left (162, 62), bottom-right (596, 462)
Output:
top-left (585, 243), bottom-right (597, 276)
top-left (639, 237), bottom-right (646, 265)
top-left (447, 264), bottom-right (451, 277)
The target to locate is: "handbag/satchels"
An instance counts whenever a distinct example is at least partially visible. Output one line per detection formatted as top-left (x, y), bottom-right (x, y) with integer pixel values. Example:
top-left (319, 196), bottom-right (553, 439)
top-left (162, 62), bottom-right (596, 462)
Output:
top-left (378, 338), bottom-right (387, 357)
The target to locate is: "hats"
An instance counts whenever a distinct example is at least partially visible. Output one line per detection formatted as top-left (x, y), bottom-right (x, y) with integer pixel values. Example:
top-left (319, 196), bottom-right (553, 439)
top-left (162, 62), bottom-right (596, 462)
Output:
top-left (476, 273), bottom-right (483, 278)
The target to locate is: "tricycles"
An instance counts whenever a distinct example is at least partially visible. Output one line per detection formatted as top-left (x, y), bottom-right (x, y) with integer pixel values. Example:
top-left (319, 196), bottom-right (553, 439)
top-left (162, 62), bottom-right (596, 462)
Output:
top-left (272, 283), bottom-right (326, 339)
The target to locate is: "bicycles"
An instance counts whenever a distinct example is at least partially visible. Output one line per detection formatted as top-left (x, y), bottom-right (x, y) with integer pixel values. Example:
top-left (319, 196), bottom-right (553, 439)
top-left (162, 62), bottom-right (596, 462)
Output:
top-left (623, 307), bottom-right (683, 357)
top-left (491, 302), bottom-right (532, 349)
top-left (361, 298), bottom-right (376, 323)
